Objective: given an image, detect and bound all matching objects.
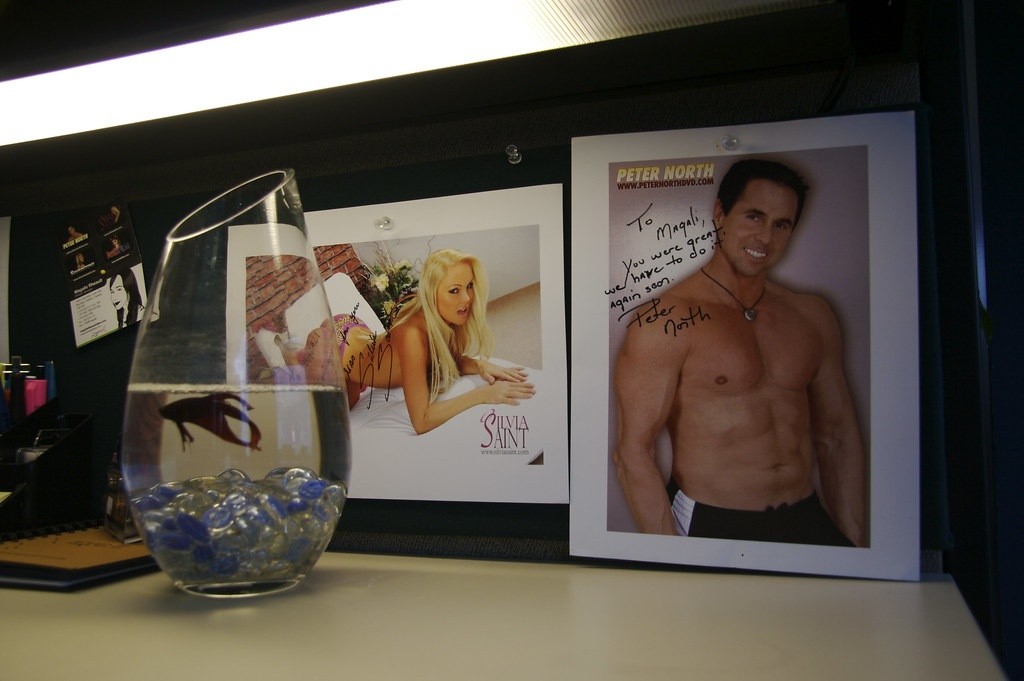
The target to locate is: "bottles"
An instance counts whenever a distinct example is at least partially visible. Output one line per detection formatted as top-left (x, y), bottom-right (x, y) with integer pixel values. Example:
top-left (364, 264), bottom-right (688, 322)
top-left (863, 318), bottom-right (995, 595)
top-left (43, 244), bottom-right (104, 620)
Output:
top-left (120, 168), bottom-right (350, 600)
top-left (102, 430), bottom-right (145, 544)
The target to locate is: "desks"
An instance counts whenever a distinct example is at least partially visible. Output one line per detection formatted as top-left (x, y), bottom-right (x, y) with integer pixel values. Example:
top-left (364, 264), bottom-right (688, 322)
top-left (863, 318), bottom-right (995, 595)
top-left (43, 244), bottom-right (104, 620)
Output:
top-left (1, 553), bottom-right (1008, 681)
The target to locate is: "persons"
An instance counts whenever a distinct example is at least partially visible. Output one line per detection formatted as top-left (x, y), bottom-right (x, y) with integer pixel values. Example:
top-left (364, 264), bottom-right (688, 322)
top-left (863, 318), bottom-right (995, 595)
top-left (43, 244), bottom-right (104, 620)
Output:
top-left (614, 162), bottom-right (868, 551)
top-left (274, 249), bottom-right (539, 435)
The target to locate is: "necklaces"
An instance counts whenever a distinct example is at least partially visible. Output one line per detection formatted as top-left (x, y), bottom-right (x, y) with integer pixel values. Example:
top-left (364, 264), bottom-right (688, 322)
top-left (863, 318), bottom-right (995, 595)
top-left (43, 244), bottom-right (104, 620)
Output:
top-left (701, 266), bottom-right (766, 320)
